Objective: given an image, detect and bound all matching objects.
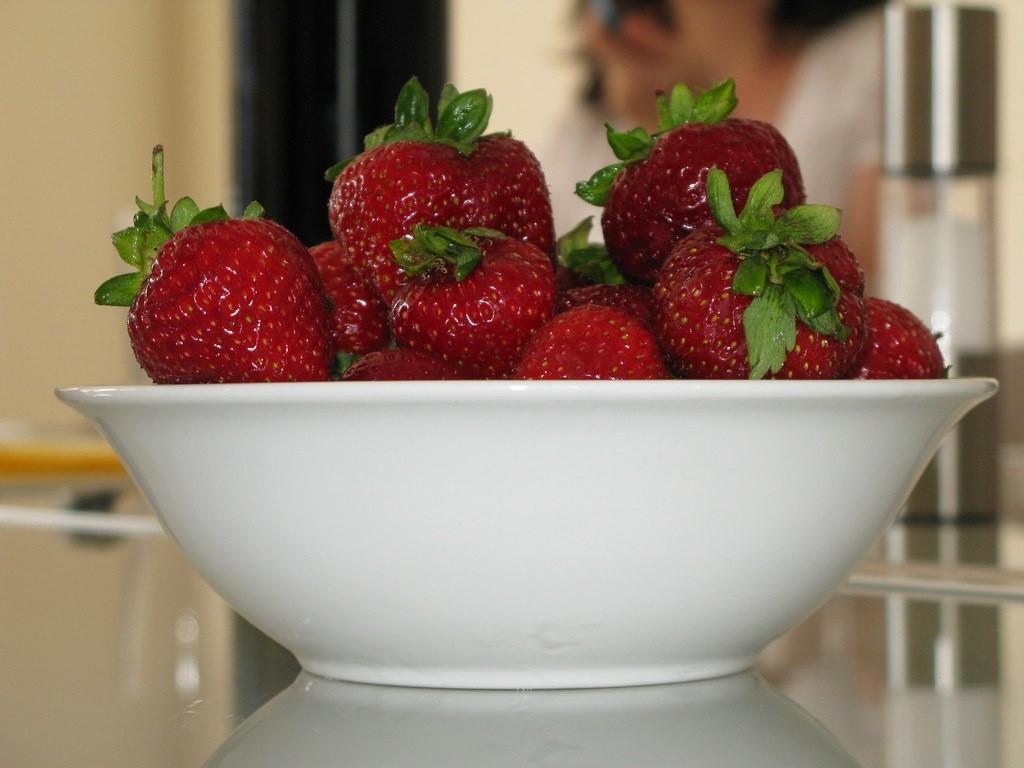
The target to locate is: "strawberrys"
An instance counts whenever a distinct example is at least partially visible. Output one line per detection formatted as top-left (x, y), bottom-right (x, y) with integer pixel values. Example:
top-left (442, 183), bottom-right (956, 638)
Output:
top-left (92, 73), bottom-right (954, 386)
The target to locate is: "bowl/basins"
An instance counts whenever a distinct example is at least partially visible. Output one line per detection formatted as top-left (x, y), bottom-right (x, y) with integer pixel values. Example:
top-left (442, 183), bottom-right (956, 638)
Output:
top-left (56, 375), bottom-right (997, 689)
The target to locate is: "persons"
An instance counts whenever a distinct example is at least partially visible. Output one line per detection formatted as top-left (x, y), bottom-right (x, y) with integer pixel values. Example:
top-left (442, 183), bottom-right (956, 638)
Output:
top-left (879, 165), bottom-right (987, 350)
top-left (542, 0), bottom-right (891, 290)
